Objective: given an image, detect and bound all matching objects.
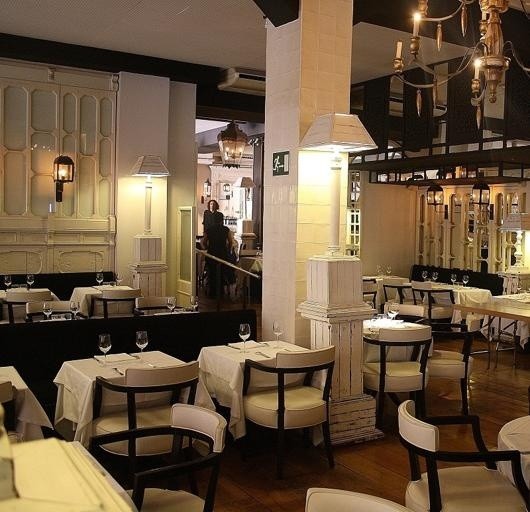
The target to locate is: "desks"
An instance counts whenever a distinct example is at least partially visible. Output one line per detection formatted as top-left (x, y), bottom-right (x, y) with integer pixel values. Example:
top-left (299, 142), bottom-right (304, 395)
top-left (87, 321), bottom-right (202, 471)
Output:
top-left (1, 285), bottom-right (143, 317)
top-left (3, 350), bottom-right (189, 512)
top-left (364, 274), bottom-right (530, 364)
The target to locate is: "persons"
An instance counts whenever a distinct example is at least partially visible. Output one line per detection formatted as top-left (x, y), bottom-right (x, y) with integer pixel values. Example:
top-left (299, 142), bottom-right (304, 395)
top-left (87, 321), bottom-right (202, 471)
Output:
top-left (200, 211), bottom-right (234, 298)
top-left (203, 200), bottom-right (223, 231)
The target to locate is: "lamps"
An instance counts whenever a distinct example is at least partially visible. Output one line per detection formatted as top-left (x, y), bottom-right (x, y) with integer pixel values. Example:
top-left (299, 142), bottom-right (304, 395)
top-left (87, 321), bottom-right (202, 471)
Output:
top-left (392, 0), bottom-right (528, 130)
top-left (235, 177), bottom-right (256, 234)
top-left (427, 184), bottom-right (491, 205)
top-left (52, 155), bottom-right (75, 203)
top-left (218, 121), bottom-right (248, 169)
top-left (128, 156), bottom-right (171, 238)
top-left (298, 113), bottom-right (378, 259)
top-left (500, 214), bottom-right (530, 273)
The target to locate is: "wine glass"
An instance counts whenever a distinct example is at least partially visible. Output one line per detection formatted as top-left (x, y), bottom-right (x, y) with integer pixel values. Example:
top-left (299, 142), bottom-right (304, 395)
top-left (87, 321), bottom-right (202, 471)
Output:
top-left (272, 319), bottom-right (285, 350)
top-left (70, 300), bottom-right (81, 320)
top-left (97, 333), bottom-right (112, 367)
top-left (26, 273), bottom-right (35, 292)
top-left (135, 330), bottom-right (148, 360)
top-left (189, 295), bottom-right (200, 312)
top-left (421, 269), bottom-right (470, 288)
top-left (165, 296), bottom-right (177, 315)
top-left (375, 262), bottom-right (394, 277)
top-left (387, 303), bottom-right (401, 324)
top-left (4, 275), bottom-right (12, 293)
top-left (238, 323), bottom-right (251, 354)
top-left (41, 301), bottom-right (54, 321)
top-left (115, 272), bottom-right (124, 289)
top-left (95, 272), bottom-right (104, 288)
